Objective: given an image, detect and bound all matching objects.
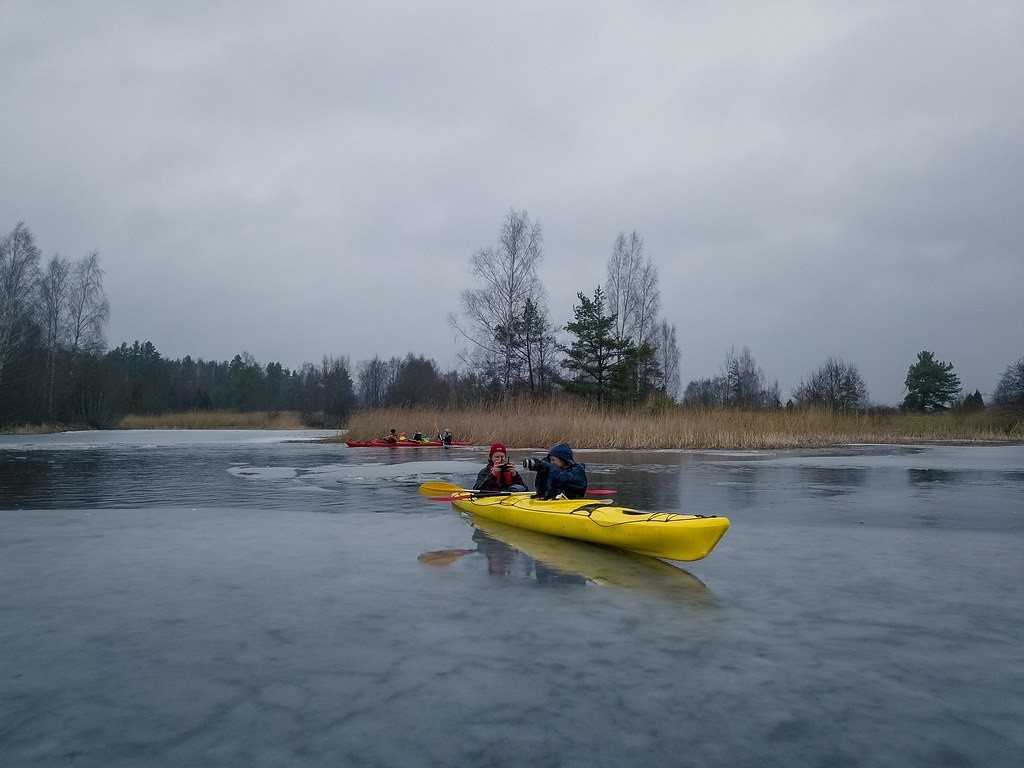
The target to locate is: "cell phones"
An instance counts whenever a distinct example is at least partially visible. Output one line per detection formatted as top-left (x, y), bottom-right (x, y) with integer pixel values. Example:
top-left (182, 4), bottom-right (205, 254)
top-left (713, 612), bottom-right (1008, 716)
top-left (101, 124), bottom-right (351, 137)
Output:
top-left (498, 464), bottom-right (510, 472)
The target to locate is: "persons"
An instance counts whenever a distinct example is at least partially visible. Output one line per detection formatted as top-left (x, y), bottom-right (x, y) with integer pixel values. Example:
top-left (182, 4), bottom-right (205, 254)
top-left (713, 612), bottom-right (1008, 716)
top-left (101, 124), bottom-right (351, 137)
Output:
top-left (534, 443), bottom-right (587, 499)
top-left (473, 443), bottom-right (528, 492)
top-left (382, 429), bottom-right (397, 443)
top-left (438, 429), bottom-right (452, 445)
top-left (412, 428), bottom-right (423, 441)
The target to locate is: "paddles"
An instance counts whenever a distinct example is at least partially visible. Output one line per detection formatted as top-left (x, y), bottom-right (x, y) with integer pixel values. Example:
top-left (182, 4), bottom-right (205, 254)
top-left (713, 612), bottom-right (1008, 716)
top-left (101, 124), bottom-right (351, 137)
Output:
top-left (419, 480), bottom-right (500, 496)
top-left (434, 422), bottom-right (449, 449)
top-left (426, 489), bottom-right (618, 502)
top-left (370, 432), bottom-right (406, 443)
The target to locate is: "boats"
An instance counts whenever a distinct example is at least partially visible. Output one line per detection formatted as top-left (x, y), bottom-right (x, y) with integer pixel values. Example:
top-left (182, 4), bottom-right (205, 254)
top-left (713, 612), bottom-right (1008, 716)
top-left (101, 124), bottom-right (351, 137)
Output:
top-left (345, 439), bottom-right (472, 447)
top-left (451, 491), bottom-right (730, 561)
top-left (460, 507), bottom-right (710, 599)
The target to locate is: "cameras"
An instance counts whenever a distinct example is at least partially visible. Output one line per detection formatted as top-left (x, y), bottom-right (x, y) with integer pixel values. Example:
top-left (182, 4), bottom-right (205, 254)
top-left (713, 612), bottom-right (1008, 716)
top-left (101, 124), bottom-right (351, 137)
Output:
top-left (523, 458), bottom-right (547, 473)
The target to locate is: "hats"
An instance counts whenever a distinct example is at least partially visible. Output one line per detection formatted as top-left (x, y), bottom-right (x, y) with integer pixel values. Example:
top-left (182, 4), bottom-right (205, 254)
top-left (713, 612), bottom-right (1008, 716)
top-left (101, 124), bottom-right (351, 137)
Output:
top-left (490, 443), bottom-right (507, 457)
top-left (445, 429), bottom-right (450, 432)
